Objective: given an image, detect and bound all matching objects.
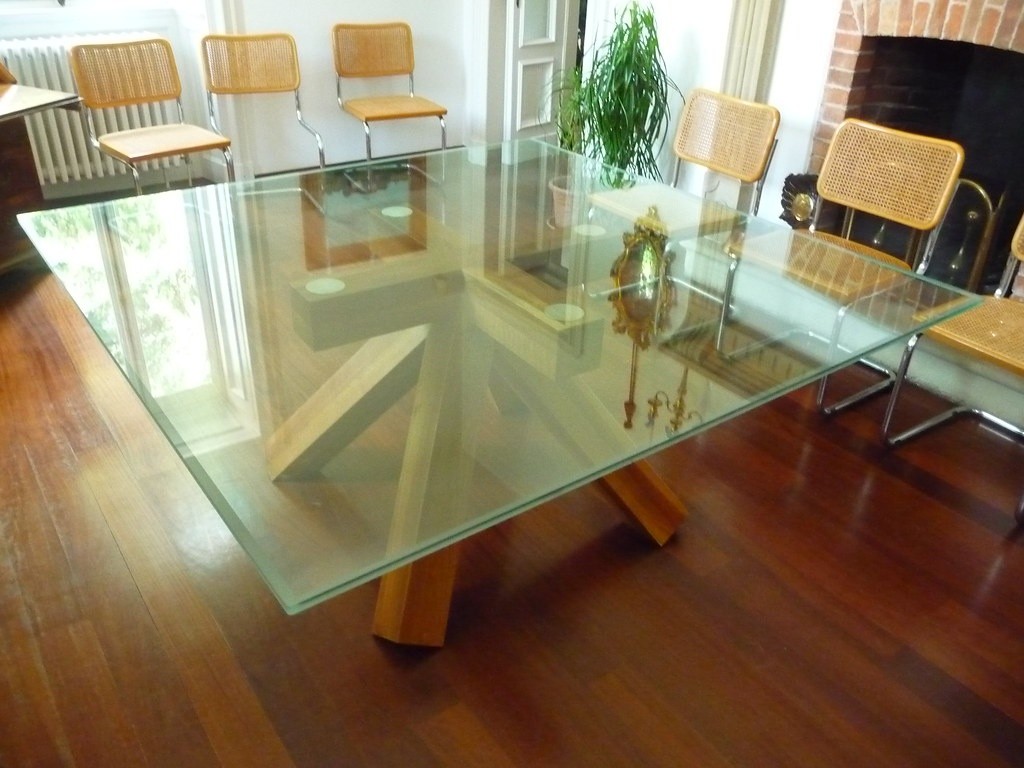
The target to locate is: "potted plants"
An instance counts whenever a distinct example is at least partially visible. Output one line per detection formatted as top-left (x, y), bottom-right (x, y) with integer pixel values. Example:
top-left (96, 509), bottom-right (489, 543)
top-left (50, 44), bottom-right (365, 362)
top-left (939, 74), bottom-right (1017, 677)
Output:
top-left (545, 2), bottom-right (686, 240)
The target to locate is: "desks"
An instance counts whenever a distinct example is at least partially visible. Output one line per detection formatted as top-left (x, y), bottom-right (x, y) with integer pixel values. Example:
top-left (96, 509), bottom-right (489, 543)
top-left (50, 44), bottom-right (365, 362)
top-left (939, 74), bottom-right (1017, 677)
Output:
top-left (16, 139), bottom-right (985, 645)
top-left (0, 81), bottom-right (86, 272)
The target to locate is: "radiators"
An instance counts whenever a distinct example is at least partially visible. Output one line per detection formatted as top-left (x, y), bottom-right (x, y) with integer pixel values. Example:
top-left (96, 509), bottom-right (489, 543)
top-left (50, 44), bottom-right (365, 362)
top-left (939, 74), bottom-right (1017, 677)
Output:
top-left (0, 32), bottom-right (184, 188)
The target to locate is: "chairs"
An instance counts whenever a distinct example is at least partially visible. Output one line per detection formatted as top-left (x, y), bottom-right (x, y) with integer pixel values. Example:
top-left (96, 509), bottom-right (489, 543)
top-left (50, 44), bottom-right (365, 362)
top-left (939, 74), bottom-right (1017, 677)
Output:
top-left (200, 32), bottom-right (328, 217)
top-left (68, 38), bottom-right (233, 246)
top-left (715, 118), bottom-right (965, 418)
top-left (576, 89), bottom-right (781, 349)
top-left (333, 20), bottom-right (444, 192)
top-left (881, 211), bottom-right (1024, 530)
